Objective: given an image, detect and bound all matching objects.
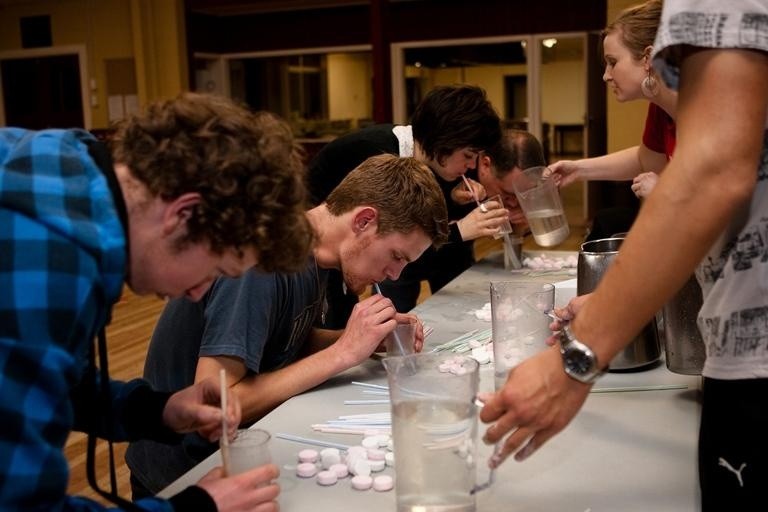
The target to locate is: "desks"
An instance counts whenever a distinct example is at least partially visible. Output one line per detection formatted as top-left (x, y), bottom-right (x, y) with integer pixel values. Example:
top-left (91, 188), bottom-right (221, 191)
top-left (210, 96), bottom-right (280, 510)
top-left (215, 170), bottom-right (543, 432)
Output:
top-left (554, 124), bottom-right (583, 155)
top-left (154, 249), bottom-right (703, 512)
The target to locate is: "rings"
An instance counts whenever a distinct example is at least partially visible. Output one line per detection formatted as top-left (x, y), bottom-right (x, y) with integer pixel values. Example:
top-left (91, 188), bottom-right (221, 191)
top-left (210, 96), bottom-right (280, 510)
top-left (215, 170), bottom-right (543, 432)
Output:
top-left (481, 203), bottom-right (487, 211)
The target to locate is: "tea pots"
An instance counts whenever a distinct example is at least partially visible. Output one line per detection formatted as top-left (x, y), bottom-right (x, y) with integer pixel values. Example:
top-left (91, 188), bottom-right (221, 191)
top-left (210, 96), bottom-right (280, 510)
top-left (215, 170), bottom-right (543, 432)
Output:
top-left (663, 273), bottom-right (706, 376)
top-left (578, 237), bottom-right (663, 371)
top-left (612, 232), bottom-right (664, 326)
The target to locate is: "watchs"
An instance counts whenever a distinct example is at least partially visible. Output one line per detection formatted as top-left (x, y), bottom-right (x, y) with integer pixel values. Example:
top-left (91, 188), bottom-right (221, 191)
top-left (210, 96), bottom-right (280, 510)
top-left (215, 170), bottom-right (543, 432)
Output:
top-left (558, 324), bottom-right (610, 387)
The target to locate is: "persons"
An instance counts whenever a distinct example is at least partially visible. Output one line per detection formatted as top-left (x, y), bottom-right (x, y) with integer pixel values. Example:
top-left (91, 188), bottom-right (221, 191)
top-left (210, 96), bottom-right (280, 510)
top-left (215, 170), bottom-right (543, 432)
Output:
top-left (432, 127), bottom-right (547, 292)
top-left (472, 0), bottom-right (768, 511)
top-left (303, 83), bottom-right (510, 330)
top-left (125, 153), bottom-right (450, 501)
top-left (543, 1), bottom-right (678, 200)
top-left (0, 90), bottom-right (323, 510)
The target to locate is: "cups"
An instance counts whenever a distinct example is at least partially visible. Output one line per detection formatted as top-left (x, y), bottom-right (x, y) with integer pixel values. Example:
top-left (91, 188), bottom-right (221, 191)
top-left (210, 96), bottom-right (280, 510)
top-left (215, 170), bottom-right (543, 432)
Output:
top-left (502, 240), bottom-right (523, 270)
top-left (380, 351), bottom-right (481, 512)
top-left (511, 164), bottom-right (571, 247)
top-left (477, 194), bottom-right (514, 243)
top-left (220, 428), bottom-right (273, 496)
top-left (487, 277), bottom-right (556, 388)
top-left (383, 315), bottom-right (418, 358)
